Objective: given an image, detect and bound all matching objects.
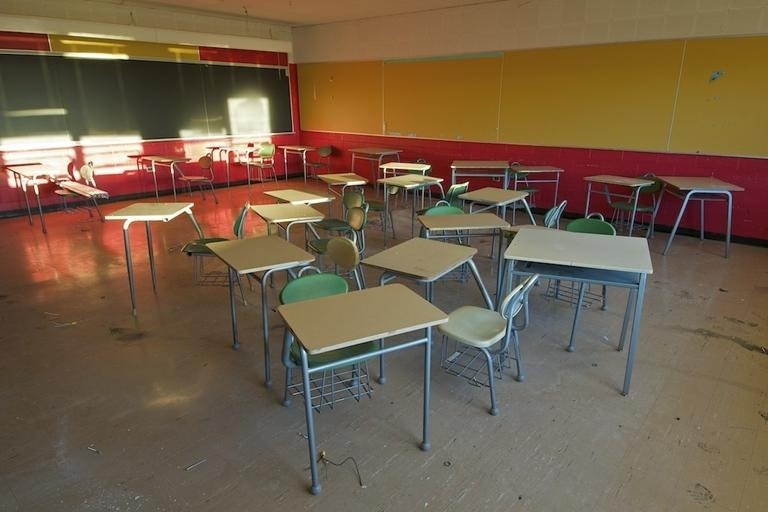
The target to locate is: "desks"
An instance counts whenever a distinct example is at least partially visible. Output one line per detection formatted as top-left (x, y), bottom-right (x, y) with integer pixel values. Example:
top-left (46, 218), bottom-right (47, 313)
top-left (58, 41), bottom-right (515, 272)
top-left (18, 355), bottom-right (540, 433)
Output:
top-left (6, 165), bottom-right (59, 233)
top-left (276, 283), bottom-right (449, 495)
top-left (657, 176), bottom-right (745, 258)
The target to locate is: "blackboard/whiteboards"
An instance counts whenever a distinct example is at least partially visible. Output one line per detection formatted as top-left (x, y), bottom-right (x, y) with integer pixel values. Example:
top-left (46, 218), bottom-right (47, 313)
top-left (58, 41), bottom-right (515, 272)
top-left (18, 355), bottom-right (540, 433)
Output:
top-left (0, 49), bottom-right (297, 153)
